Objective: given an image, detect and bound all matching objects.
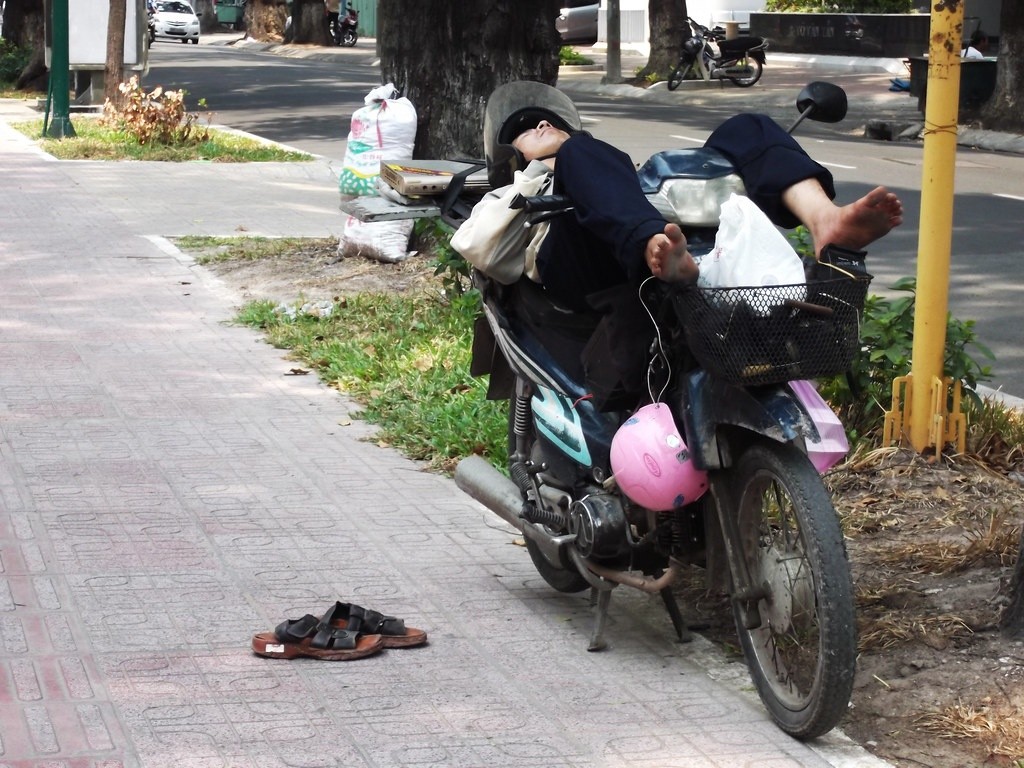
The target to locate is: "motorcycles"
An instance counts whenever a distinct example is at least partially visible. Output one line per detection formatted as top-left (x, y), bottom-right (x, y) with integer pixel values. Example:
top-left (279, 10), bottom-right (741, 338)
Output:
top-left (440, 81), bottom-right (875, 741)
top-left (330, 2), bottom-right (360, 47)
top-left (147, 1), bottom-right (159, 49)
top-left (667, 17), bottom-right (770, 92)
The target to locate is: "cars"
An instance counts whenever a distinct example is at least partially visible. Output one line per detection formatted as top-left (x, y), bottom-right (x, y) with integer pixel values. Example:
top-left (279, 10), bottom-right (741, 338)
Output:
top-left (154, 0), bottom-right (202, 44)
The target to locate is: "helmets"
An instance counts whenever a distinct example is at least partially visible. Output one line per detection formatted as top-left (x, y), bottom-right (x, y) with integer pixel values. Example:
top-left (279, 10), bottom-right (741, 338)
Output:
top-left (610, 403), bottom-right (710, 512)
top-left (486, 105), bottom-right (578, 188)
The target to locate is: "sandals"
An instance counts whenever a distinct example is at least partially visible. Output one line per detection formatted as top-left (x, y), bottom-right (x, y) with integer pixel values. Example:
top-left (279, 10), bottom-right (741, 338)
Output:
top-left (252, 601), bottom-right (428, 661)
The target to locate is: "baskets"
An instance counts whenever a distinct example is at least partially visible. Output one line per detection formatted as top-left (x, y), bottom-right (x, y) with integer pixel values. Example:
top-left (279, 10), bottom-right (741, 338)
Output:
top-left (663, 247), bottom-right (874, 386)
top-left (681, 36), bottom-right (702, 56)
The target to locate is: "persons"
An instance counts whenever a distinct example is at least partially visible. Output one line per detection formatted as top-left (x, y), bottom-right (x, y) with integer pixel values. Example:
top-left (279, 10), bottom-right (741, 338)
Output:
top-left (483, 81), bottom-right (903, 316)
top-left (324, 0), bottom-right (342, 46)
